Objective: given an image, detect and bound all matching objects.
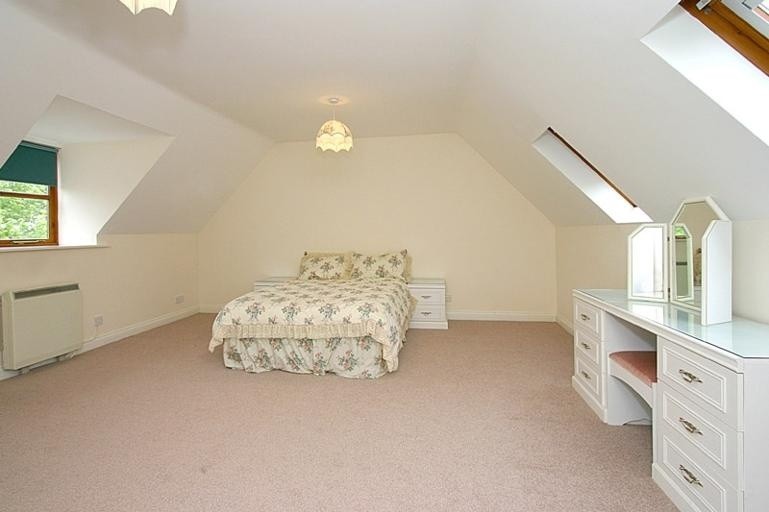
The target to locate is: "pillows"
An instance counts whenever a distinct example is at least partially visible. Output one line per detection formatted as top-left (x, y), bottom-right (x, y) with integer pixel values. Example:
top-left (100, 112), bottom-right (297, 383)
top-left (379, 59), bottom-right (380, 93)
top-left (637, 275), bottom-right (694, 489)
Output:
top-left (299, 250), bottom-right (407, 282)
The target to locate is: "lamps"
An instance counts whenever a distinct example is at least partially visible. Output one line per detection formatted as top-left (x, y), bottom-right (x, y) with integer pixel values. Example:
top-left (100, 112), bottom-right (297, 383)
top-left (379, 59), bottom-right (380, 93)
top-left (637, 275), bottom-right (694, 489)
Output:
top-left (315, 98), bottom-right (354, 153)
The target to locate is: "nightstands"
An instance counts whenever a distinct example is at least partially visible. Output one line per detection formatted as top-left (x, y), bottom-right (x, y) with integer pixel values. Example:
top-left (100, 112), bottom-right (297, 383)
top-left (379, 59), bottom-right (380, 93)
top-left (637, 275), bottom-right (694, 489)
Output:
top-left (254, 277), bottom-right (298, 291)
top-left (406, 279), bottom-right (449, 330)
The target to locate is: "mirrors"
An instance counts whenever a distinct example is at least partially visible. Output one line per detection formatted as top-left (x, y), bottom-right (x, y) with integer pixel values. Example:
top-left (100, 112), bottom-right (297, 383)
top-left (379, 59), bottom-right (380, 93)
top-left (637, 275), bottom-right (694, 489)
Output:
top-left (672, 222), bottom-right (694, 302)
top-left (626, 196), bottom-right (734, 325)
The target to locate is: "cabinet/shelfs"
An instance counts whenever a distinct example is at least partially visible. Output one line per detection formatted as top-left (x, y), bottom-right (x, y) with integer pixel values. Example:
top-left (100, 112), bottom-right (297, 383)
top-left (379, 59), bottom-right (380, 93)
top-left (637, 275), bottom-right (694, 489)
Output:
top-left (570, 286), bottom-right (768, 511)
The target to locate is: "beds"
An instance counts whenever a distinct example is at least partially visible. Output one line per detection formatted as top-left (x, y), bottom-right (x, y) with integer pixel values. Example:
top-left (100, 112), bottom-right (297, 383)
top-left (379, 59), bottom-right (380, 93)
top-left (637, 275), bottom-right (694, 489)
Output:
top-left (223, 281), bottom-right (408, 378)
